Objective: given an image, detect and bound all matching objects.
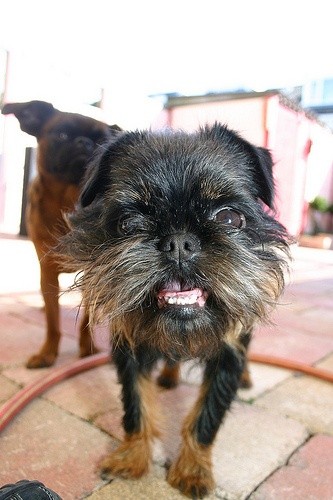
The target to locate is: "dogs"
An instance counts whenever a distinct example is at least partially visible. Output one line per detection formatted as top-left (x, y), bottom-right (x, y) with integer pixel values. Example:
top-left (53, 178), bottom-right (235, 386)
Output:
top-left (36, 120), bottom-right (297, 500)
top-left (2, 100), bottom-right (123, 369)
